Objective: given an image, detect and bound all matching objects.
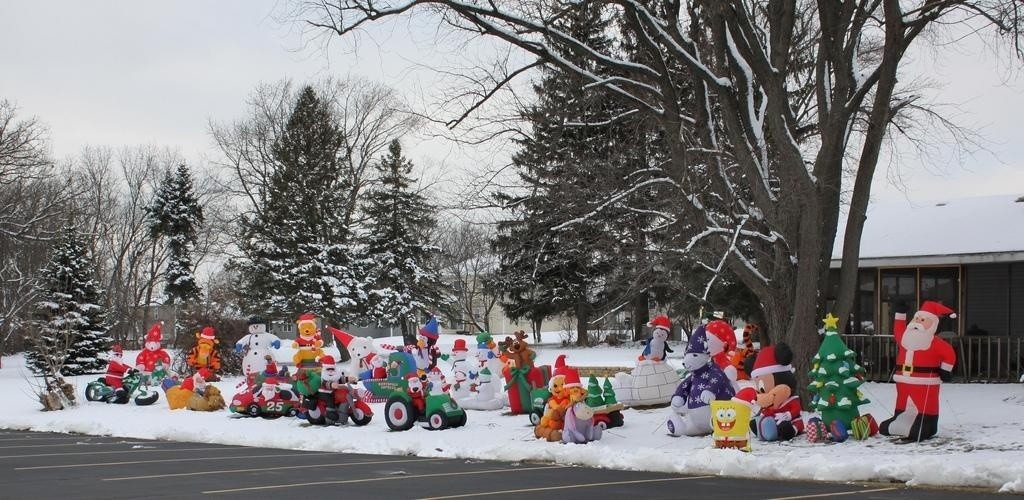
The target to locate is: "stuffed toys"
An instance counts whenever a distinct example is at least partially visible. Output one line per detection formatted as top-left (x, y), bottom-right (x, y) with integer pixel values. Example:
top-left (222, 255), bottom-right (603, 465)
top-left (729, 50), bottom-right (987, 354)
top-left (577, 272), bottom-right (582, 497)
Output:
top-left (277, 377), bottom-right (301, 402)
top-left (535, 355), bottom-right (571, 442)
top-left (379, 342), bottom-right (441, 366)
top-left (540, 386), bottom-right (587, 430)
top-left (179, 367), bottom-right (211, 397)
top-left (749, 341), bottom-right (805, 441)
top-left (416, 369), bottom-right (428, 390)
top-left (292, 314), bottom-right (323, 368)
top-left (705, 320), bottom-right (757, 404)
top-left (161, 378), bottom-right (181, 393)
top-left (473, 332), bottom-right (504, 378)
top-left (400, 372), bottom-right (425, 416)
top-left (261, 362), bottom-right (277, 377)
top-left (638, 317), bottom-right (674, 361)
top-left (187, 393), bottom-right (212, 411)
top-left (411, 313), bottom-right (439, 373)
top-left (725, 323), bottom-right (757, 380)
top-left (204, 385), bottom-right (221, 401)
top-left (427, 368), bottom-right (451, 396)
top-left (450, 369), bottom-right (472, 399)
top-left (709, 400), bottom-right (752, 452)
top-left (666, 319), bottom-right (736, 437)
top-left (136, 320), bottom-right (180, 385)
top-left (208, 396), bottom-right (225, 410)
top-left (504, 330), bottom-right (536, 368)
top-left (166, 385), bottom-right (192, 409)
top-left (457, 367), bottom-right (503, 410)
top-left (561, 399), bottom-right (602, 445)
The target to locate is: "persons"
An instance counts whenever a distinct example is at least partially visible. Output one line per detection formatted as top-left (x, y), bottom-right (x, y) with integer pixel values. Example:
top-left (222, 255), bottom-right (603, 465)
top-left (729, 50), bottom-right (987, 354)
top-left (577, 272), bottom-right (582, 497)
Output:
top-left (254, 378), bottom-right (281, 402)
top-left (452, 339), bottom-right (479, 380)
top-left (235, 316), bottom-right (281, 393)
top-left (315, 355), bottom-right (358, 425)
top-left (324, 324), bottom-right (400, 379)
top-left (186, 327), bottom-right (221, 382)
top-left (105, 342), bottom-right (139, 397)
top-left (565, 368), bottom-right (582, 398)
top-left (878, 296), bottom-right (956, 441)
